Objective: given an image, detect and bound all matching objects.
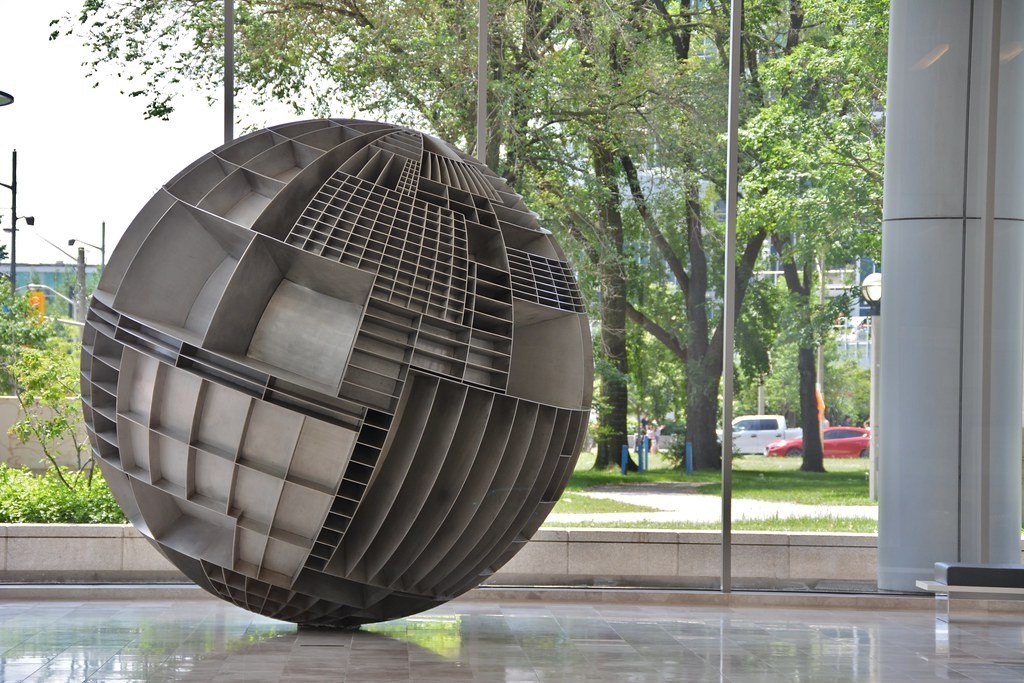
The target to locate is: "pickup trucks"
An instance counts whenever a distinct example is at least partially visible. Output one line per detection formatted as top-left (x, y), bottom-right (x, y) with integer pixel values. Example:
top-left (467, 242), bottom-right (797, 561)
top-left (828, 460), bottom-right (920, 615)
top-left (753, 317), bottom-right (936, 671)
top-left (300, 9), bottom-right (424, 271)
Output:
top-left (715, 415), bottom-right (802, 456)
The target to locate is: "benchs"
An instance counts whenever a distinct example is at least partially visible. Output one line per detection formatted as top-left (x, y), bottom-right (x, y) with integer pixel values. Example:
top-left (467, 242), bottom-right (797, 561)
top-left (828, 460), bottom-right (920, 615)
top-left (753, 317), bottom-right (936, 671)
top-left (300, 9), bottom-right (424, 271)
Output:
top-left (916, 562), bottom-right (1024, 623)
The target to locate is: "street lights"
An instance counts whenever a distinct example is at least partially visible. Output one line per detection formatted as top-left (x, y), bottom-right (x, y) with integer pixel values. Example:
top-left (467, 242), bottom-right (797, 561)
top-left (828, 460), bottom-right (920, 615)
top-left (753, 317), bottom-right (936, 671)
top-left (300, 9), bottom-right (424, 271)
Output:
top-left (68, 223), bottom-right (107, 266)
top-left (862, 270), bottom-right (880, 504)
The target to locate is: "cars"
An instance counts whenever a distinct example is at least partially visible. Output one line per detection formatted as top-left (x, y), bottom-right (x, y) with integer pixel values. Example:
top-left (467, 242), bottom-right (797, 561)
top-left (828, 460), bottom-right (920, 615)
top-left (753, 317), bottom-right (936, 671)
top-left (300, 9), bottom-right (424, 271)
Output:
top-left (764, 427), bottom-right (870, 458)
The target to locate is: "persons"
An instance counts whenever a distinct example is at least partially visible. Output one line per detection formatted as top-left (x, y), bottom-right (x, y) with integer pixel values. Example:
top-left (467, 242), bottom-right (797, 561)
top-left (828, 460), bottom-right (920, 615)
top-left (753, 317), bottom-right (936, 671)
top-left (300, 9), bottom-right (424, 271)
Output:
top-left (841, 415), bottom-right (852, 426)
top-left (640, 412), bottom-right (664, 452)
top-left (856, 419), bottom-right (862, 427)
top-left (823, 415), bottom-right (829, 429)
top-left (860, 420), bottom-right (871, 437)
